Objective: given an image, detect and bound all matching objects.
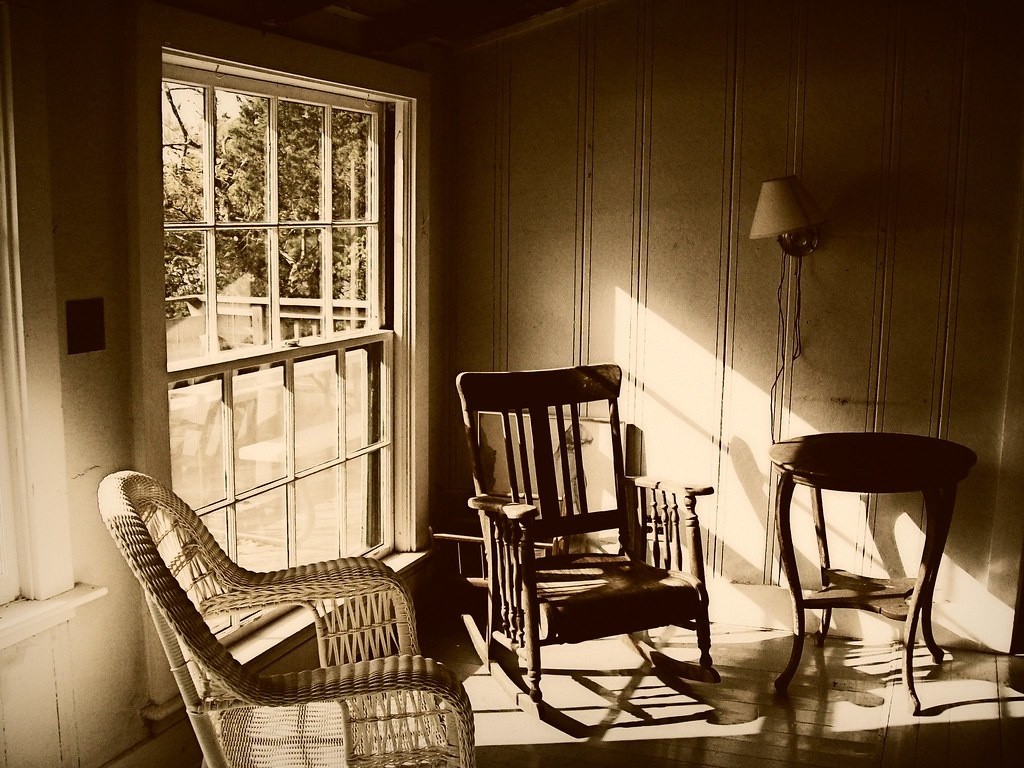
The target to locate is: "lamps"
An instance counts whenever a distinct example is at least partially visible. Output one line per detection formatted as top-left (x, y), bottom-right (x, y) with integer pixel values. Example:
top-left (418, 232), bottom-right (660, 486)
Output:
top-left (748, 175), bottom-right (831, 259)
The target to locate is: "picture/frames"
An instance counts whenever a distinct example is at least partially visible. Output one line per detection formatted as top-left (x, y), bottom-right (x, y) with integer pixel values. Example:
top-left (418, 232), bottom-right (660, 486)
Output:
top-left (476, 411), bottom-right (630, 557)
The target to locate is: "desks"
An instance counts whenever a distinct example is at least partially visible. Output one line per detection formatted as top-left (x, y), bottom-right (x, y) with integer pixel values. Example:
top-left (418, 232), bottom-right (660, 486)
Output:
top-left (768, 432), bottom-right (975, 719)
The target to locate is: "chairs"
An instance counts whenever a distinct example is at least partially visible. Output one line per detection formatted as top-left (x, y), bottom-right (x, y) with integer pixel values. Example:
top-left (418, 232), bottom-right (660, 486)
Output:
top-left (455, 363), bottom-right (721, 720)
top-left (97, 470), bottom-right (477, 768)
